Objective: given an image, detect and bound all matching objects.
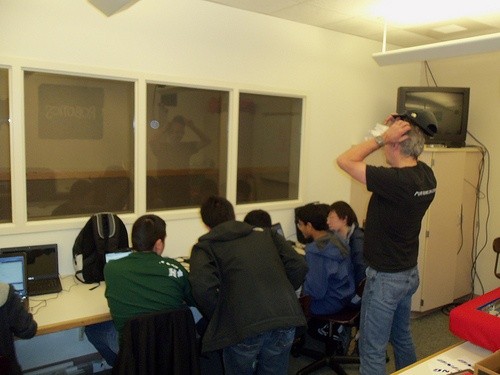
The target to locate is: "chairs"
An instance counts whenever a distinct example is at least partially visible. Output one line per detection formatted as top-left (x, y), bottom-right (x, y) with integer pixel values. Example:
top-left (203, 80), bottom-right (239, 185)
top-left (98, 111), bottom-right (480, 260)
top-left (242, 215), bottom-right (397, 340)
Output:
top-left (113, 311), bottom-right (202, 375)
top-left (293, 278), bottom-right (389, 375)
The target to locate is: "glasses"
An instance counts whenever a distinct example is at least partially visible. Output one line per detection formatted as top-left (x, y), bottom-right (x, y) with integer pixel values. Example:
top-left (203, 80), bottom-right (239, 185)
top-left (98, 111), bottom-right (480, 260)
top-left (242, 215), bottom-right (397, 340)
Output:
top-left (297, 223), bottom-right (307, 228)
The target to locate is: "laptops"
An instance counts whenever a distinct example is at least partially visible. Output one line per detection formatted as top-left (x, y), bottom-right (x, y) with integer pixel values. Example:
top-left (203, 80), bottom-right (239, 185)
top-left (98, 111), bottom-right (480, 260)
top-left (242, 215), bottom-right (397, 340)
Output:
top-left (0, 252), bottom-right (27, 305)
top-left (271, 223), bottom-right (284, 239)
top-left (1, 244), bottom-right (62, 296)
top-left (103, 248), bottom-right (133, 265)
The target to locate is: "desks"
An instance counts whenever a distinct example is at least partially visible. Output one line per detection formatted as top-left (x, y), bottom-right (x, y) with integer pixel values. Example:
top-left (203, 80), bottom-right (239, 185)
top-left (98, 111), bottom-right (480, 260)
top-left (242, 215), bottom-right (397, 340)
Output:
top-left (1, 165), bottom-right (260, 219)
top-left (13, 243), bottom-right (308, 341)
top-left (390, 339), bottom-right (492, 375)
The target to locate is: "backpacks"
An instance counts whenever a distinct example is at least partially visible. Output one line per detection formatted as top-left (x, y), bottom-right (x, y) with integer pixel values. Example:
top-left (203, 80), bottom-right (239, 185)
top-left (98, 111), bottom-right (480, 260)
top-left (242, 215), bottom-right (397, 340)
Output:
top-left (73, 211), bottom-right (130, 291)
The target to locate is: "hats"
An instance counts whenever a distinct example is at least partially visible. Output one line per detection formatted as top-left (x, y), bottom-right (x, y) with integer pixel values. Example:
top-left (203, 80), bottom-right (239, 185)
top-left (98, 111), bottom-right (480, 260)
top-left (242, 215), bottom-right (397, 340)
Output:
top-left (392, 107), bottom-right (439, 137)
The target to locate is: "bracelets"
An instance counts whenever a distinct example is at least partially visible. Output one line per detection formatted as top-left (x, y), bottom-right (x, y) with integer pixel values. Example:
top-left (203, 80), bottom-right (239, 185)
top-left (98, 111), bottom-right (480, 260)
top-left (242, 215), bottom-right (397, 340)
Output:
top-left (375, 135), bottom-right (385, 147)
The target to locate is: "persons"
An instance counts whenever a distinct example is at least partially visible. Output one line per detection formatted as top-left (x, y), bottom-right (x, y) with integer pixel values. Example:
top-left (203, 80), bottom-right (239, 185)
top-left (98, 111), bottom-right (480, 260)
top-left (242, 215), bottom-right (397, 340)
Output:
top-left (48, 164), bottom-right (252, 217)
top-left (291, 202), bottom-right (362, 358)
top-left (336, 106), bottom-right (438, 375)
top-left (244, 208), bottom-right (275, 229)
top-left (189, 197), bottom-right (309, 375)
top-left (0, 276), bottom-right (39, 375)
top-left (325, 200), bottom-right (374, 294)
top-left (84, 213), bottom-right (190, 375)
top-left (147, 115), bottom-right (213, 173)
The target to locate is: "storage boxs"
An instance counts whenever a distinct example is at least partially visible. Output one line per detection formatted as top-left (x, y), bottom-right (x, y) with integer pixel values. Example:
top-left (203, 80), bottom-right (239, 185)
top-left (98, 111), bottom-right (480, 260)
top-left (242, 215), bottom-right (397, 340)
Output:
top-left (474, 350), bottom-right (500, 375)
top-left (448, 288), bottom-right (500, 351)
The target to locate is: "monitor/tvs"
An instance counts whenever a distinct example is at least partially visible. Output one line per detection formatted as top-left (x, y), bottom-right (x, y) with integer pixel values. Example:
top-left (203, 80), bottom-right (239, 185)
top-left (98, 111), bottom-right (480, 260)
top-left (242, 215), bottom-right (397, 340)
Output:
top-left (397, 87), bottom-right (470, 149)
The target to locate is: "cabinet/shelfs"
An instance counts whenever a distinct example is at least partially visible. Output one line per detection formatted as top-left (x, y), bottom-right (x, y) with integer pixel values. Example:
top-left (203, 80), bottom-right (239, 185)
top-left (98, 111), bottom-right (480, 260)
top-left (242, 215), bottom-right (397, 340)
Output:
top-left (349, 140), bottom-right (483, 319)
